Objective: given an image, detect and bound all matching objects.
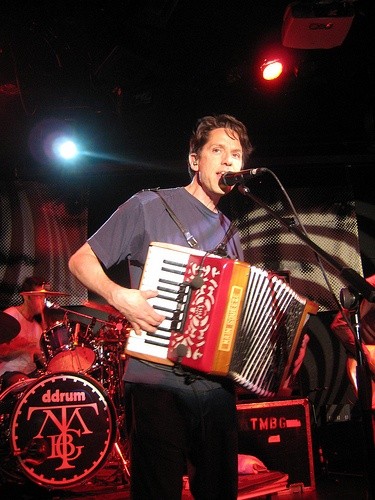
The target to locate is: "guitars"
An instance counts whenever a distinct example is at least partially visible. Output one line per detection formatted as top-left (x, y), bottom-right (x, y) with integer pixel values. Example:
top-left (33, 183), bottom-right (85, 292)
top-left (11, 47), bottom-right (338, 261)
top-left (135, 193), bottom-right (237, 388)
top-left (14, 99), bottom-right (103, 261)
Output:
top-left (346, 343), bottom-right (375, 411)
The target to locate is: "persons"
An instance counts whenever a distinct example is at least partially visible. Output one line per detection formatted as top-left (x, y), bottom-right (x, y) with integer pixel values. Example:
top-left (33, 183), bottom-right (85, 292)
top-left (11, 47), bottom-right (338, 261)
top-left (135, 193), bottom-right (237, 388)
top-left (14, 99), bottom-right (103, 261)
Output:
top-left (330, 273), bottom-right (375, 396)
top-left (0, 277), bottom-right (53, 402)
top-left (67, 114), bottom-right (311, 500)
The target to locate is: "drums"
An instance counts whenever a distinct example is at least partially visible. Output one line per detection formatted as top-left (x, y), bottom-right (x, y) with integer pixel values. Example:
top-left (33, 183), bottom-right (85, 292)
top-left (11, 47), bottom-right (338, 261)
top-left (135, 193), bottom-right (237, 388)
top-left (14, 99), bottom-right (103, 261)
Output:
top-left (0, 370), bottom-right (117, 488)
top-left (39, 319), bottom-right (125, 372)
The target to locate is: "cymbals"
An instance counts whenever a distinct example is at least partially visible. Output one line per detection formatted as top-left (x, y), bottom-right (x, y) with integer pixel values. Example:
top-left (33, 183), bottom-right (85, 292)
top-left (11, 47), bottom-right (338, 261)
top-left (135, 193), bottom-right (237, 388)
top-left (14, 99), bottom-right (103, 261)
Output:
top-left (19, 287), bottom-right (72, 298)
top-left (80, 299), bottom-right (129, 319)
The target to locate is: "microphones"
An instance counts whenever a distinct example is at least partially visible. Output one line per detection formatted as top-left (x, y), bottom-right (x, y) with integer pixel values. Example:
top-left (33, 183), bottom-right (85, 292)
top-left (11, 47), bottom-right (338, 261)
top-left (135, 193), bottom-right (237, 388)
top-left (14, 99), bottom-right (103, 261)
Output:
top-left (221, 168), bottom-right (267, 186)
top-left (45, 301), bottom-right (60, 310)
